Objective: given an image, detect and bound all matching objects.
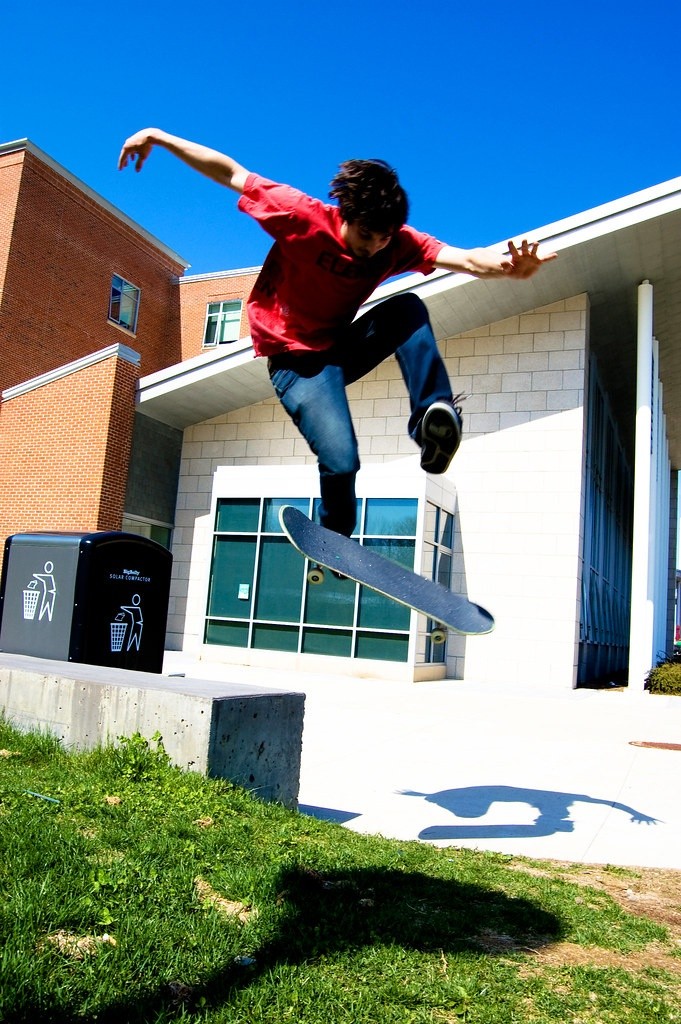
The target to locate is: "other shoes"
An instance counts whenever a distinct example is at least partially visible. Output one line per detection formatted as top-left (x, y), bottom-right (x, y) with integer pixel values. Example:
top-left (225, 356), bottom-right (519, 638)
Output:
top-left (421, 390), bottom-right (473, 474)
top-left (329, 570), bottom-right (347, 580)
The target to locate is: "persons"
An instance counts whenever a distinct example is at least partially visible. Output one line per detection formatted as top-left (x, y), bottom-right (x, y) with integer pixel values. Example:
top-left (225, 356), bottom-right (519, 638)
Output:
top-left (118, 128), bottom-right (559, 581)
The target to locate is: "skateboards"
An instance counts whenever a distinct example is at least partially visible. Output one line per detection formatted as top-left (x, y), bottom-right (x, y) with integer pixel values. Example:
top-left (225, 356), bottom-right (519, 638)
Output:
top-left (278, 505), bottom-right (494, 644)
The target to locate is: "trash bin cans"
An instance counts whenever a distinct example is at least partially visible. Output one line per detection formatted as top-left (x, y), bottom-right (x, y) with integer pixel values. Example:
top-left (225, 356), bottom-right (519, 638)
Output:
top-left (0, 530), bottom-right (173, 673)
